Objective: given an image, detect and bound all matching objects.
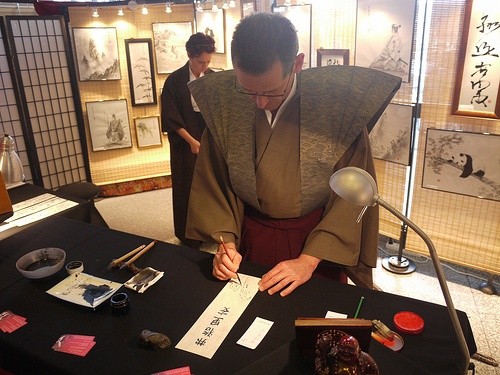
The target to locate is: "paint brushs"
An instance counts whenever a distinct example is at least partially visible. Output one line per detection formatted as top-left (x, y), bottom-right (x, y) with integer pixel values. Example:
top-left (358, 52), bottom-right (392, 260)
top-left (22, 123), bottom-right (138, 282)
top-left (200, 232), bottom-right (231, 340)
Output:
top-left (120, 240), bottom-right (155, 269)
top-left (111, 244), bottom-right (145, 268)
top-left (219, 236), bottom-right (242, 284)
top-left (354, 296), bottom-right (365, 320)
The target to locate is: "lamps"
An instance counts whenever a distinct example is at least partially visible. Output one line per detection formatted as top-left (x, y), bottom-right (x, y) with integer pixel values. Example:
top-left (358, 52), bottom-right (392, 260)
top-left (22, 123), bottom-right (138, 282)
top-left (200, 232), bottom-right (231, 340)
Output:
top-left (0, 134), bottom-right (25, 183)
top-left (118, 0), bottom-right (124, 16)
top-left (166, 0), bottom-right (237, 12)
top-left (92, 5), bottom-right (100, 17)
top-left (328, 166), bottom-right (471, 372)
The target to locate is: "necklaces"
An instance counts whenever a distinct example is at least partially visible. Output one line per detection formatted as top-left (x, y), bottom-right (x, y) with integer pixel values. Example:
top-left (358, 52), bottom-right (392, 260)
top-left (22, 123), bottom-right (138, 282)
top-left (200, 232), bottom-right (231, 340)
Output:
top-left (334, 356), bottom-right (338, 375)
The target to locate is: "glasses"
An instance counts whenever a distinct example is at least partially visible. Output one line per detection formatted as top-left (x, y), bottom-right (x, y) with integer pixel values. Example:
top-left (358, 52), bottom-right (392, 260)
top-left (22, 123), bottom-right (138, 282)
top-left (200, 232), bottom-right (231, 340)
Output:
top-left (235, 60), bottom-right (294, 97)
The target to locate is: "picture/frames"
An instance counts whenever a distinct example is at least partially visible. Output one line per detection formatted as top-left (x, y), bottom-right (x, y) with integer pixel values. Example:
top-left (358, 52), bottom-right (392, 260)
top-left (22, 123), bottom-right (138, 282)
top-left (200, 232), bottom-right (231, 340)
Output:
top-left (125, 38), bottom-right (158, 106)
top-left (151, 21), bottom-right (193, 75)
top-left (451, 0), bottom-right (500, 120)
top-left (133, 115), bottom-right (164, 149)
top-left (316, 49), bottom-right (349, 67)
top-left (272, 4), bottom-right (312, 70)
top-left (421, 128), bottom-right (500, 204)
top-left (194, 8), bottom-right (225, 54)
top-left (368, 100), bottom-right (415, 166)
top-left (72, 26), bottom-right (121, 82)
top-left (240, 0), bottom-right (256, 18)
top-left (85, 99), bottom-right (133, 152)
top-left (353, 0), bottom-right (417, 82)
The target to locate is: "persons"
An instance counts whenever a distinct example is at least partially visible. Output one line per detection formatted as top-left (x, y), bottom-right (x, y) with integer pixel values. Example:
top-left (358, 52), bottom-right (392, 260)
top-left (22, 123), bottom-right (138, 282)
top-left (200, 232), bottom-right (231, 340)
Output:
top-left (162, 33), bottom-right (216, 247)
top-left (185, 10), bottom-right (402, 297)
top-left (318, 336), bottom-right (379, 375)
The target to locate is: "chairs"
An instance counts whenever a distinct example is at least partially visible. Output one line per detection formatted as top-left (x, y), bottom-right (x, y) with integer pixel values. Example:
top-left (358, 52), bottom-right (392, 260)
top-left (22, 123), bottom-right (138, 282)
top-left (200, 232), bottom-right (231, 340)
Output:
top-left (55, 183), bottom-right (100, 223)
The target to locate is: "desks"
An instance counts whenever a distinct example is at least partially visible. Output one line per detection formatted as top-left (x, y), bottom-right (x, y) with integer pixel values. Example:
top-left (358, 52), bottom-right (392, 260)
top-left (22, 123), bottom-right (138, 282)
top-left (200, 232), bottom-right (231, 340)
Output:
top-left (0, 183), bottom-right (477, 375)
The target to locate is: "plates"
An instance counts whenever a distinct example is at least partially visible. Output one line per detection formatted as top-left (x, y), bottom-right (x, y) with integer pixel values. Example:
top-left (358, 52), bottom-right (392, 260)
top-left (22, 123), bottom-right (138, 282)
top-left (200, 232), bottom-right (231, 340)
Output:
top-left (15, 247), bottom-right (66, 279)
